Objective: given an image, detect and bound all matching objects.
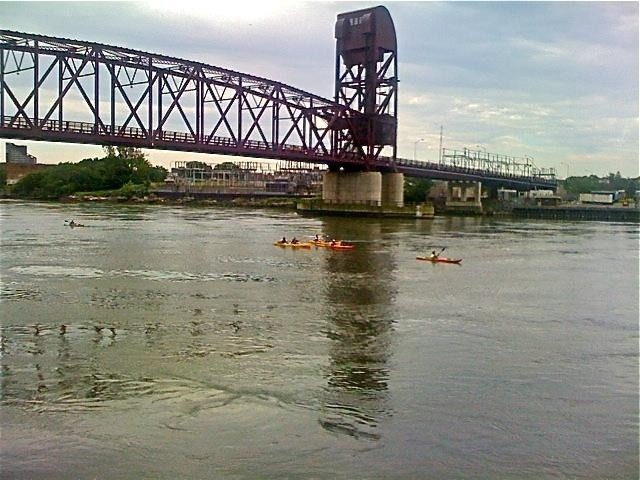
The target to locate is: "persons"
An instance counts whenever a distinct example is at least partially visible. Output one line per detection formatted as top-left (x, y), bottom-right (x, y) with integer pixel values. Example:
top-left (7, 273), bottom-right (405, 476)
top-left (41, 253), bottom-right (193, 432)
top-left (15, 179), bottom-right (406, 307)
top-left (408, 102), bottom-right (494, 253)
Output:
top-left (291, 237), bottom-right (299, 244)
top-left (431, 250), bottom-right (440, 258)
top-left (314, 234), bottom-right (319, 241)
top-left (281, 237), bottom-right (287, 243)
top-left (331, 239), bottom-right (336, 245)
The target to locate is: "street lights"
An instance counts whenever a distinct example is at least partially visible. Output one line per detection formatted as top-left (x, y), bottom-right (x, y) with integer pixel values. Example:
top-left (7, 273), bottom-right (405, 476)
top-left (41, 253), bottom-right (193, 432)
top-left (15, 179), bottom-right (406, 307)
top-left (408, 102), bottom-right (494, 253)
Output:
top-left (523, 153), bottom-right (533, 177)
top-left (414, 137), bottom-right (423, 164)
top-left (476, 144), bottom-right (487, 171)
top-left (560, 161), bottom-right (569, 177)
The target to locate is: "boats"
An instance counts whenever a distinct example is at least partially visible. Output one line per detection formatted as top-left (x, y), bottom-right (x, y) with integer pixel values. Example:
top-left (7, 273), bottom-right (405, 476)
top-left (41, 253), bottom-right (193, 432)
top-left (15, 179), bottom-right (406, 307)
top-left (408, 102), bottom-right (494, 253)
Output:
top-left (416, 255), bottom-right (462, 263)
top-left (273, 241), bottom-right (311, 249)
top-left (64, 223), bottom-right (84, 228)
top-left (315, 243), bottom-right (353, 250)
top-left (308, 240), bottom-right (341, 245)
